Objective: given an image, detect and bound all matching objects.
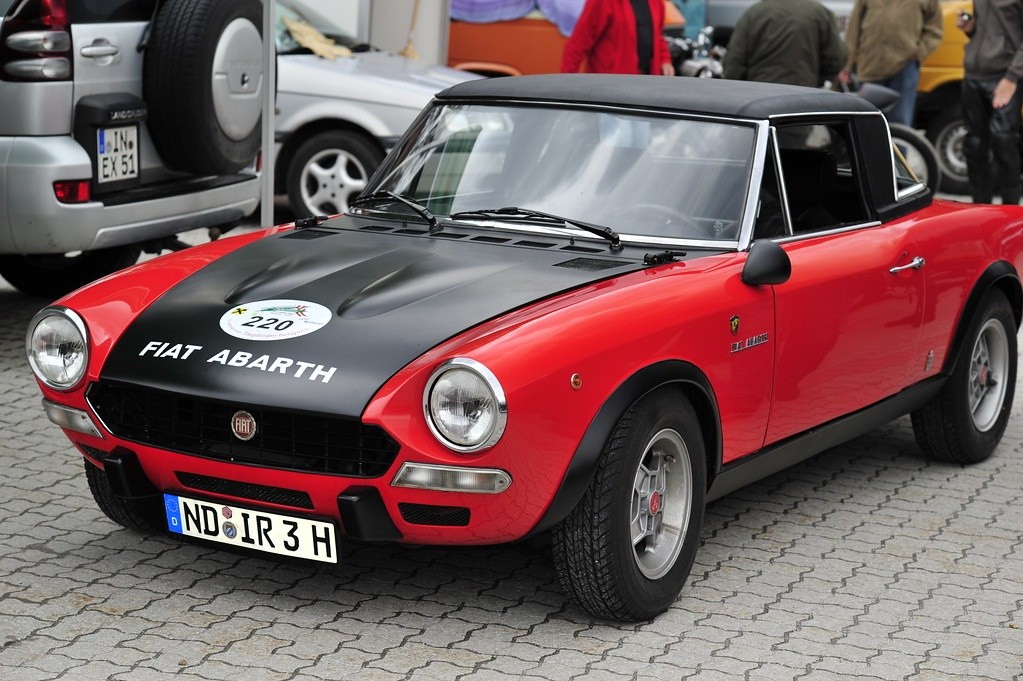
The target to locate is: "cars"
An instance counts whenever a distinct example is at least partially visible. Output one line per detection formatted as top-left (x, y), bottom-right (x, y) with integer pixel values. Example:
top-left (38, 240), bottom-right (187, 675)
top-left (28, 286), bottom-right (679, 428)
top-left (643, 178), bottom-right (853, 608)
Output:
top-left (271, 1), bottom-right (516, 225)
top-left (25, 72), bottom-right (1022, 627)
top-left (909, 0), bottom-right (997, 195)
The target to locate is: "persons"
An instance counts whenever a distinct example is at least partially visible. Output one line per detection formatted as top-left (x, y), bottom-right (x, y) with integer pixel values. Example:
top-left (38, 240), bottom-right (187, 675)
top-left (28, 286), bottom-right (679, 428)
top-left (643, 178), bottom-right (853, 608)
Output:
top-left (955, 0), bottom-right (1023, 205)
top-left (837, 0), bottom-right (943, 176)
top-left (722, 0), bottom-right (849, 147)
top-left (562, 0), bottom-right (675, 149)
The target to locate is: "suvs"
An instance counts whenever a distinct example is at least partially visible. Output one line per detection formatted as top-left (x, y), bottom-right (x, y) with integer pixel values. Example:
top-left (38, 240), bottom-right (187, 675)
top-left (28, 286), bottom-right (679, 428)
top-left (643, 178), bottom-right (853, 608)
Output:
top-left (0, 0), bottom-right (277, 299)
